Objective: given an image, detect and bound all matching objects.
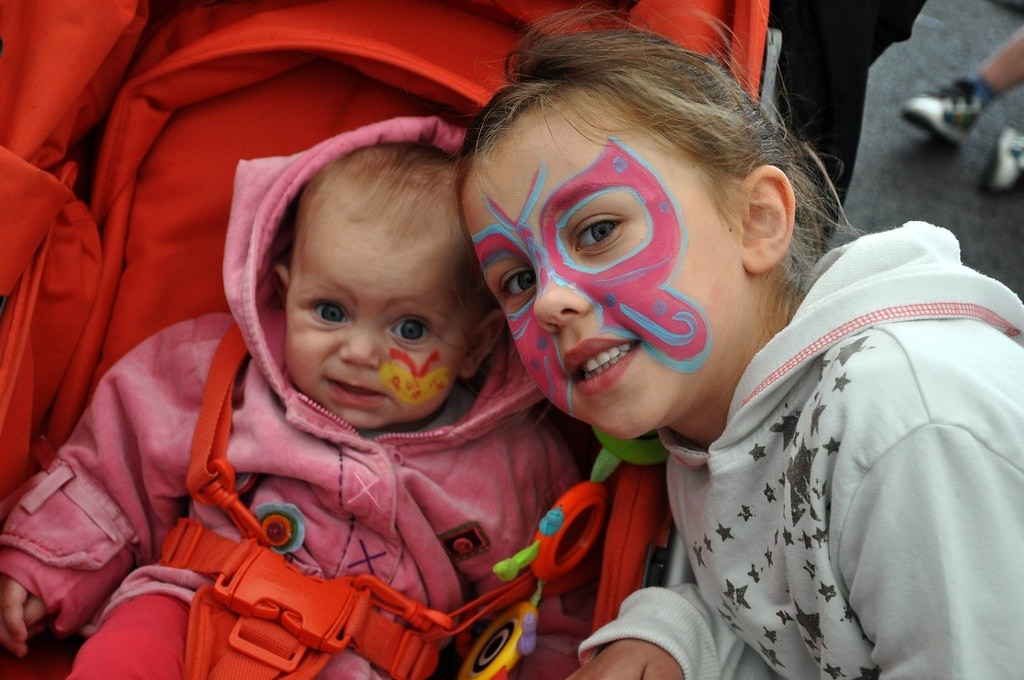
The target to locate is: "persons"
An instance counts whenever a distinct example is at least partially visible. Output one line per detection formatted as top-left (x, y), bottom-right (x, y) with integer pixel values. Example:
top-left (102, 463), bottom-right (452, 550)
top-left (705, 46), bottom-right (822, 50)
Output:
top-left (460, 0), bottom-right (1024, 680)
top-left (0, 113), bottom-right (593, 680)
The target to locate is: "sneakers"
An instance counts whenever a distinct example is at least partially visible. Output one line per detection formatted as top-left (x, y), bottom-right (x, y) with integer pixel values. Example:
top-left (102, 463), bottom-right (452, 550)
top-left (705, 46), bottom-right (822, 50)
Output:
top-left (902, 76), bottom-right (984, 147)
top-left (982, 126), bottom-right (1024, 192)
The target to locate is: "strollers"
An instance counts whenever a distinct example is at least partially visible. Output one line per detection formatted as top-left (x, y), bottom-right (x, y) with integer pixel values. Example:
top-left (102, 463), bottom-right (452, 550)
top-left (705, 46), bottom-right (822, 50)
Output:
top-left (0, 0), bottom-right (782, 678)
